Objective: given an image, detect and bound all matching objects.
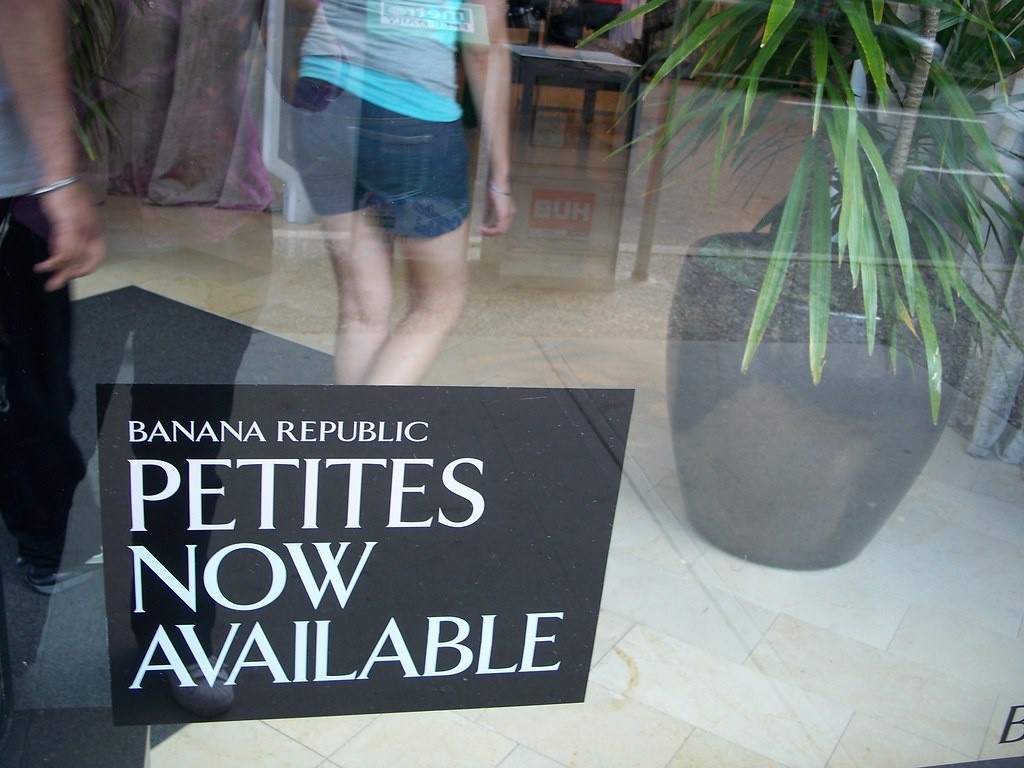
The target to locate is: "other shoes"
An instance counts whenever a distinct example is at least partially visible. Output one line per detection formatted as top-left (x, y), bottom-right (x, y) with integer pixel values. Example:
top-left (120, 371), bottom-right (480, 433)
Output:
top-left (25, 544), bottom-right (104, 595)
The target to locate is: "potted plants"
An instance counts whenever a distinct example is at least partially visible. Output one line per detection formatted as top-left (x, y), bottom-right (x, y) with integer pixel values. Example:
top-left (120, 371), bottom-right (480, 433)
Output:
top-left (571, 0), bottom-right (1024, 573)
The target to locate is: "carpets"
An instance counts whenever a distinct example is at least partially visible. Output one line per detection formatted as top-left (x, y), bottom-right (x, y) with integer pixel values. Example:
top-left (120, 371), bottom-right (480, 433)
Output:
top-left (1, 285), bottom-right (337, 768)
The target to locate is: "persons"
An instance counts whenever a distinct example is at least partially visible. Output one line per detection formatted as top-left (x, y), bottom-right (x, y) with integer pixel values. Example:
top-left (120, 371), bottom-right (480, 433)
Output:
top-left (71, 0), bottom-right (283, 749)
top-left (0, 1), bottom-right (108, 592)
top-left (294, 0), bottom-right (516, 386)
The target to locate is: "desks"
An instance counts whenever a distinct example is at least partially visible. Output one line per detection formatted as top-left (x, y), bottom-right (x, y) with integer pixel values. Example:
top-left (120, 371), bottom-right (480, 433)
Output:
top-left (498, 42), bottom-right (643, 151)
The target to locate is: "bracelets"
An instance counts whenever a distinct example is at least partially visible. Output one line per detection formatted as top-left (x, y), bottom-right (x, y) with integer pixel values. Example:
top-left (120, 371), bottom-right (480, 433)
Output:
top-left (488, 183), bottom-right (513, 197)
top-left (33, 177), bottom-right (79, 198)
